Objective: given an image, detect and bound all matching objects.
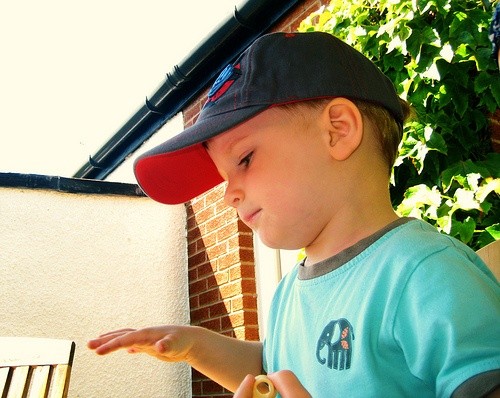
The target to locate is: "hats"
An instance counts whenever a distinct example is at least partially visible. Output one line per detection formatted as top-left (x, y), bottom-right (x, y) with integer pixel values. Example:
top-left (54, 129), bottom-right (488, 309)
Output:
top-left (132, 31), bottom-right (406, 206)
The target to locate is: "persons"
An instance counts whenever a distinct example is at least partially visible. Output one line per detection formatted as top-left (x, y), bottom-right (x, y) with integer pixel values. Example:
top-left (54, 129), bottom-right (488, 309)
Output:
top-left (88, 31), bottom-right (500, 398)
